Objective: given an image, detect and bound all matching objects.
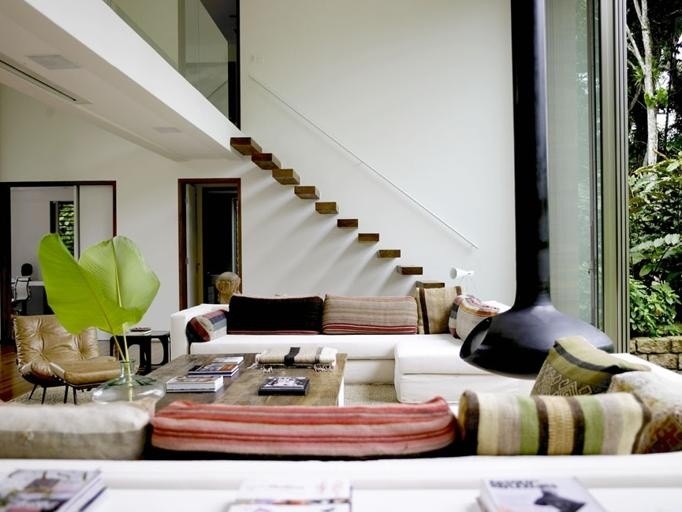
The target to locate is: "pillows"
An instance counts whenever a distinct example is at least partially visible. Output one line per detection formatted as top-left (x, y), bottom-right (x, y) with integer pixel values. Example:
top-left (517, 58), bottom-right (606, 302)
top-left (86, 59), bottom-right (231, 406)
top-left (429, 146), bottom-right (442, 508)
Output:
top-left (448, 296), bottom-right (480, 337)
top-left (413, 287), bottom-right (463, 335)
top-left (186, 309), bottom-right (227, 344)
top-left (529, 335), bottom-right (644, 397)
top-left (457, 295), bottom-right (501, 340)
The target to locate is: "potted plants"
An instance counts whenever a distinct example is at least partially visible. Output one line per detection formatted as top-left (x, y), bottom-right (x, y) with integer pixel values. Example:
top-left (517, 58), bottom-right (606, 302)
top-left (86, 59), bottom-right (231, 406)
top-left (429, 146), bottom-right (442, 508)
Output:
top-left (37, 230), bottom-right (167, 413)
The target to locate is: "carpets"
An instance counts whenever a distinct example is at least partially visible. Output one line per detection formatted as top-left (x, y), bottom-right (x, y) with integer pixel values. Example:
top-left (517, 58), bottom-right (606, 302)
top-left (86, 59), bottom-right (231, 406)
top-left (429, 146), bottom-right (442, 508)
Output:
top-left (5, 333), bottom-right (171, 410)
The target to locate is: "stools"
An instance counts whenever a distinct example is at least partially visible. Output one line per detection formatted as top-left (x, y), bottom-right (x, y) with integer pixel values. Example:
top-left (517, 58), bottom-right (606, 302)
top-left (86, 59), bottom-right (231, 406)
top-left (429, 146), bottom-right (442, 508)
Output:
top-left (50, 361), bottom-right (123, 403)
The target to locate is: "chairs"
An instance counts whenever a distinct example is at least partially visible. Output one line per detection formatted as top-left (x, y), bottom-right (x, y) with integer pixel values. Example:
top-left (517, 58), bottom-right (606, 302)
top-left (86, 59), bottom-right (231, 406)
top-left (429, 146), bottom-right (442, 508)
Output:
top-left (11, 275), bottom-right (32, 305)
top-left (10, 314), bottom-right (114, 404)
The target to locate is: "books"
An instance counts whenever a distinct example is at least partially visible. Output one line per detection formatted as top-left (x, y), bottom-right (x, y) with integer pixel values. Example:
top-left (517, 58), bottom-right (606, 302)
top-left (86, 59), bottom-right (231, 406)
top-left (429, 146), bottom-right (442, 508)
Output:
top-left (257, 376), bottom-right (310, 396)
top-left (167, 375), bottom-right (224, 392)
top-left (0, 468), bottom-right (107, 512)
top-left (212, 356), bottom-right (244, 363)
top-left (476, 476), bottom-right (609, 511)
top-left (224, 481), bottom-right (350, 512)
top-left (187, 363), bottom-right (238, 377)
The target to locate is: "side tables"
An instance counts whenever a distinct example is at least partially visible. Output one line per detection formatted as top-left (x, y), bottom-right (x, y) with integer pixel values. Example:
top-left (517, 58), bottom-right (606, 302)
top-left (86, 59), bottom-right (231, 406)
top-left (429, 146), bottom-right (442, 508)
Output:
top-left (109, 330), bottom-right (168, 374)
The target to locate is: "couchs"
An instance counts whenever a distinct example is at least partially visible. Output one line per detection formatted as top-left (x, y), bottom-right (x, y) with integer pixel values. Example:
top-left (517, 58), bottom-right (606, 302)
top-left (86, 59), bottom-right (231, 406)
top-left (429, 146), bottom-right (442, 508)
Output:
top-left (0, 351), bottom-right (682, 489)
top-left (170, 301), bottom-right (536, 401)
